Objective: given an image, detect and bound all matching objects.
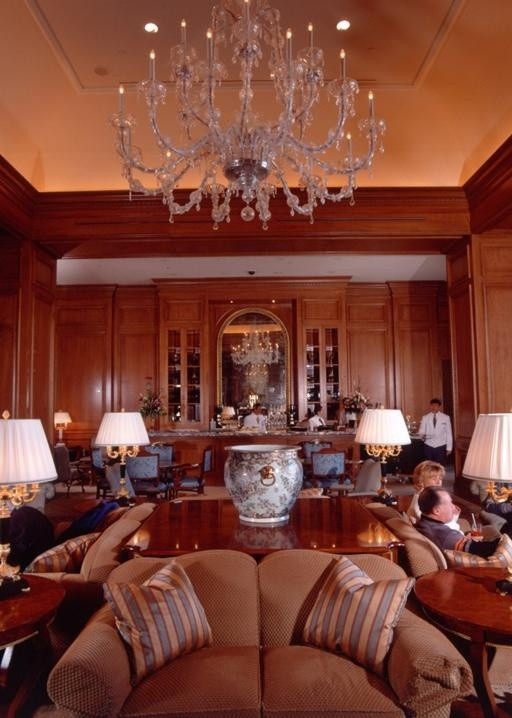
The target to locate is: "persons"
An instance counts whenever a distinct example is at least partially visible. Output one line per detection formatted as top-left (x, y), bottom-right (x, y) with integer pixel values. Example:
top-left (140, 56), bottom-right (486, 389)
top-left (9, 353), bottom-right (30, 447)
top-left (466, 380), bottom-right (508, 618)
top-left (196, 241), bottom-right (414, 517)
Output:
top-left (243, 403), bottom-right (267, 431)
top-left (412, 485), bottom-right (465, 553)
top-left (418, 397), bottom-right (453, 462)
top-left (406, 460), bottom-right (464, 536)
top-left (307, 404), bottom-right (326, 432)
top-left (0, 485), bottom-right (54, 602)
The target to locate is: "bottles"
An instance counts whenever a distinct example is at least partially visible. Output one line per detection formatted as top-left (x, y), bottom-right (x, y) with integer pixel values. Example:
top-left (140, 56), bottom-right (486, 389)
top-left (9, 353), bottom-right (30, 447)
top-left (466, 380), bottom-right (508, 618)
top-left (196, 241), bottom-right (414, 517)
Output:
top-left (214, 406), bottom-right (222, 428)
top-left (289, 404), bottom-right (296, 426)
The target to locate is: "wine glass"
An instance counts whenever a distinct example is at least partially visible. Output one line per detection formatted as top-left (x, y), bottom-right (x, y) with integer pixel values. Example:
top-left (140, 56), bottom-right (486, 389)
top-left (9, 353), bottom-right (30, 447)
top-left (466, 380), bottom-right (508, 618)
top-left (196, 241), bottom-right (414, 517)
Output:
top-left (471, 523), bottom-right (481, 537)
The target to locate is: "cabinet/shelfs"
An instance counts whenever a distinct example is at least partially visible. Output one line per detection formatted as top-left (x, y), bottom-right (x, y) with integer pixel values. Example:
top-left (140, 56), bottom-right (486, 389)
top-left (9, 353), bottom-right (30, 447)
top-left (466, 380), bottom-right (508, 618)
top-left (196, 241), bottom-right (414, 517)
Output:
top-left (156, 323), bottom-right (214, 427)
top-left (297, 319), bottom-right (349, 420)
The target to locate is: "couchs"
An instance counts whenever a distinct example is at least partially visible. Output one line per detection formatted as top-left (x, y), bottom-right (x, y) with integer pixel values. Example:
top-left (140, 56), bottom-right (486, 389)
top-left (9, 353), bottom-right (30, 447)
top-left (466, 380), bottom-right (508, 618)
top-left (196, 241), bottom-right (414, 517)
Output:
top-left (0, 497), bottom-right (512, 717)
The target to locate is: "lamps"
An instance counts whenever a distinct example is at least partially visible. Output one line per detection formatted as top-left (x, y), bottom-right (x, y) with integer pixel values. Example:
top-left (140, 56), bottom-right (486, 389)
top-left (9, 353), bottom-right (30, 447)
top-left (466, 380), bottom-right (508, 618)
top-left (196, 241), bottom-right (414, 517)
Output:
top-left (54, 409), bottom-right (74, 446)
top-left (460, 412), bottom-right (512, 523)
top-left (91, 406), bottom-right (152, 505)
top-left (221, 405), bottom-right (236, 425)
top-left (1, 418), bottom-right (58, 584)
top-left (232, 319), bottom-right (280, 393)
top-left (110, 2), bottom-right (387, 235)
top-left (354, 406), bottom-right (413, 505)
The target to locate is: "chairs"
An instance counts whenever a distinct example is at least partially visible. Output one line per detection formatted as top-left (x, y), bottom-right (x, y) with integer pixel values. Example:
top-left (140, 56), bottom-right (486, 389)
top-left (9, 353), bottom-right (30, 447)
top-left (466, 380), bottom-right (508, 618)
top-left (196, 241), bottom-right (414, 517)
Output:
top-left (54, 440), bottom-right (419, 504)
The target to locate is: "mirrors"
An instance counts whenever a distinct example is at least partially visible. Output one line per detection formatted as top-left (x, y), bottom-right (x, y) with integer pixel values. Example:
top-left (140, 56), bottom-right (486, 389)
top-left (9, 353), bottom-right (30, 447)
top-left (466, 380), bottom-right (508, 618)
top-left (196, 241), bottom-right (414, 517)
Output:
top-left (215, 307), bottom-right (289, 420)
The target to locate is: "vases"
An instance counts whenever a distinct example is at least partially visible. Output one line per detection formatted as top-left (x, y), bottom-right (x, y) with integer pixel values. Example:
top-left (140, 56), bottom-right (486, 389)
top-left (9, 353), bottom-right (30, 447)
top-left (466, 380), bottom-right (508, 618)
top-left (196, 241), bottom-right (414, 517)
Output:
top-left (147, 415), bottom-right (158, 429)
top-left (357, 412), bottom-right (365, 423)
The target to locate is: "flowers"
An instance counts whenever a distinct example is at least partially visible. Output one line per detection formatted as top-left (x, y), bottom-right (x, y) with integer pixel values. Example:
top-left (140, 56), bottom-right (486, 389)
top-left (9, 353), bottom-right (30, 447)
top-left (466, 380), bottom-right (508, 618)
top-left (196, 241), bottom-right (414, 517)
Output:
top-left (341, 392), bottom-right (372, 411)
top-left (139, 384), bottom-right (167, 415)
